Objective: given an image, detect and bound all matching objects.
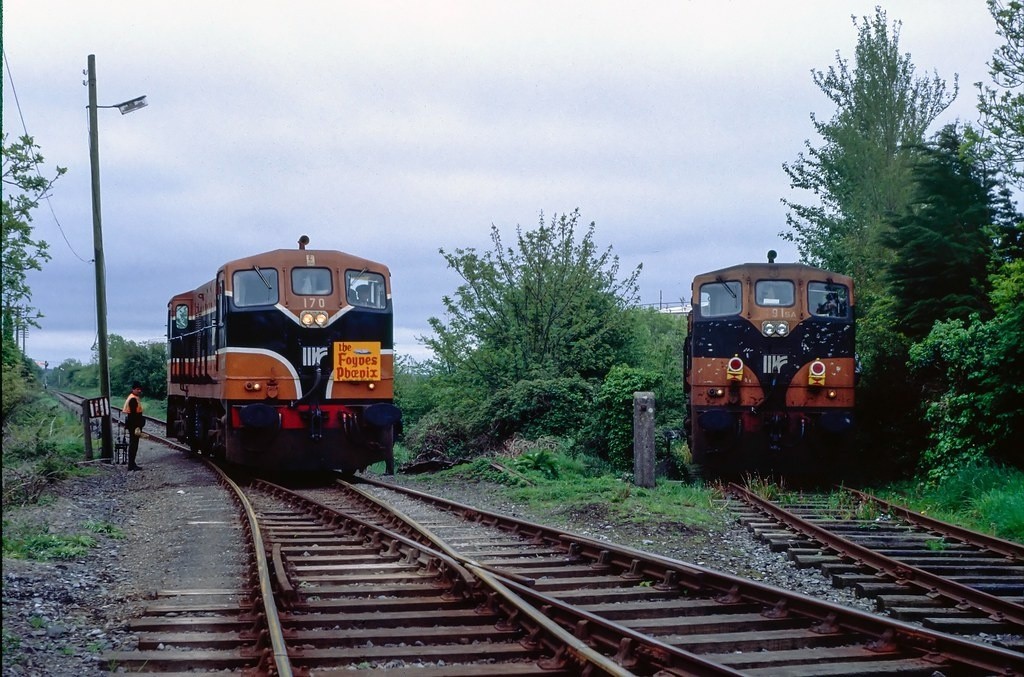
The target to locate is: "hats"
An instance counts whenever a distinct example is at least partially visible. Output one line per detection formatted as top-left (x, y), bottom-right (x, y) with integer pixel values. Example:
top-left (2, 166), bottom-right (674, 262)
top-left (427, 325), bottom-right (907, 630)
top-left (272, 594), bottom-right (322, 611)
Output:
top-left (356, 285), bottom-right (372, 291)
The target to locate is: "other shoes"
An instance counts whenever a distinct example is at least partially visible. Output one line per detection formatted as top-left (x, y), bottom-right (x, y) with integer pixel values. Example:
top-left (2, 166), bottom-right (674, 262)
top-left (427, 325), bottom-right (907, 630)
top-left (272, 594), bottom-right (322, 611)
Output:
top-left (128, 466), bottom-right (142, 471)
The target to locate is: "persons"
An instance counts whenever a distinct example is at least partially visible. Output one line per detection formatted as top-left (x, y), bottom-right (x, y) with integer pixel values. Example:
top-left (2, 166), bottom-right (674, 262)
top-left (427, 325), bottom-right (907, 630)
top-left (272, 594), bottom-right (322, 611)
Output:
top-left (348, 282), bottom-right (374, 307)
top-left (122, 383), bottom-right (146, 471)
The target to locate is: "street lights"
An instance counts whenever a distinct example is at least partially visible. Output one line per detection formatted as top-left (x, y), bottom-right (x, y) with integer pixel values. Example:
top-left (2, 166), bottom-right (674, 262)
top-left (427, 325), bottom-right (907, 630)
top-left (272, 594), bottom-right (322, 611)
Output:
top-left (82, 53), bottom-right (150, 462)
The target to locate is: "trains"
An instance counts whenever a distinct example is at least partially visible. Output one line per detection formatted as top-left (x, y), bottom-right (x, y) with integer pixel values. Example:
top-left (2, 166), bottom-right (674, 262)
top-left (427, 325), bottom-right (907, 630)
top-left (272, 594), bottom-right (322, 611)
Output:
top-left (682, 250), bottom-right (855, 473)
top-left (166, 235), bottom-right (403, 476)
